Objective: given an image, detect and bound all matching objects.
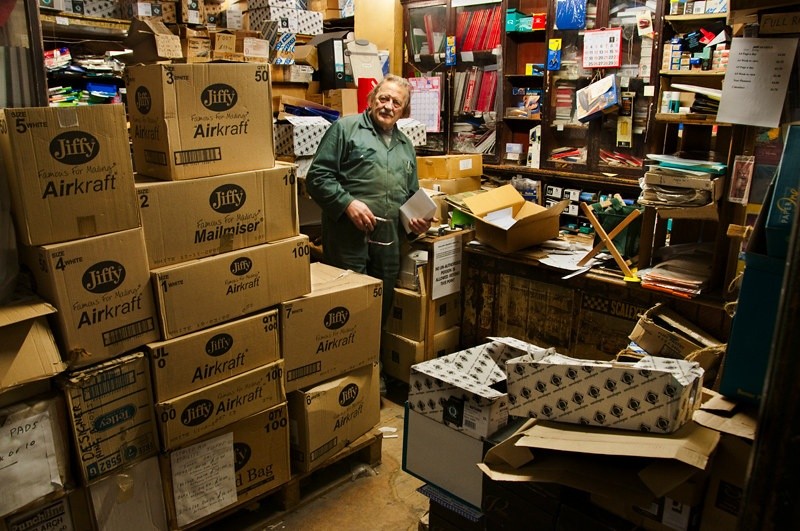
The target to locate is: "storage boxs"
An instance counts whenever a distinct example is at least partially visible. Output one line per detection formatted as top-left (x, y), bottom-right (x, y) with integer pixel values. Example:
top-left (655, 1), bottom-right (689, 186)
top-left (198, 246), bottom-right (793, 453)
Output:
top-left (443, 185), bottom-right (570, 254)
top-left (400, 334), bottom-right (756, 502)
top-left (386, 284), bottom-right (464, 338)
top-left (386, 328), bottom-right (457, 384)
top-left (0, 0), bottom-right (389, 528)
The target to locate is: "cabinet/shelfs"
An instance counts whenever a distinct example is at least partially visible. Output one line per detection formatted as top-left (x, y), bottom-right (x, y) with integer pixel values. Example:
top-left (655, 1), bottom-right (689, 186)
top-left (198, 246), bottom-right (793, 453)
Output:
top-left (501, 0), bottom-right (658, 186)
top-left (656, 1), bottom-right (799, 322)
top-left (400, 1), bottom-right (501, 164)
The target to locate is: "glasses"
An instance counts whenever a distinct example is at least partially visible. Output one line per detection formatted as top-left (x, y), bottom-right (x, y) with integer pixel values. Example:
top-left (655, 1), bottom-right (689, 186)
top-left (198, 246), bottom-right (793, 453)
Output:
top-left (363, 217), bottom-right (395, 245)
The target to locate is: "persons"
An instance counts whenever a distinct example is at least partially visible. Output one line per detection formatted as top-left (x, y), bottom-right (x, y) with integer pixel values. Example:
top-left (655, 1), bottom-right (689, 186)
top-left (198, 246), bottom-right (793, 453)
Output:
top-left (304, 73), bottom-right (439, 397)
